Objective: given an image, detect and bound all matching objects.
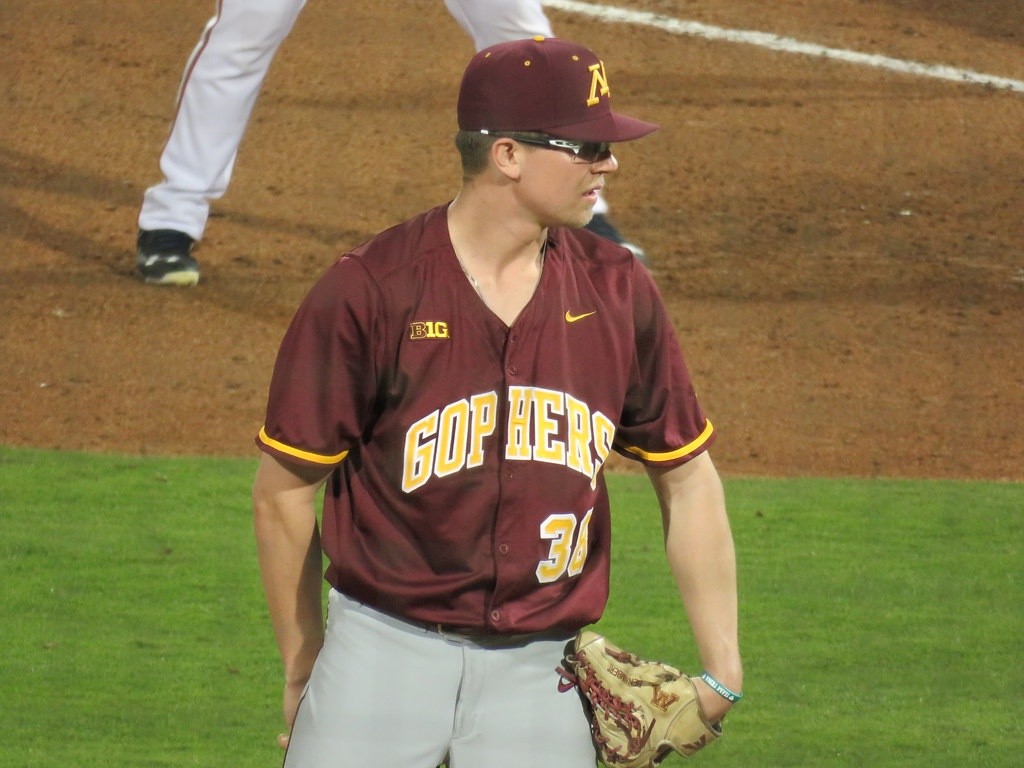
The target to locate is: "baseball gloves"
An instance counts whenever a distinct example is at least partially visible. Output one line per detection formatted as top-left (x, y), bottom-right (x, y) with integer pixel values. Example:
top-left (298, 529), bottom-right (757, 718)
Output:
top-left (555, 631), bottom-right (724, 768)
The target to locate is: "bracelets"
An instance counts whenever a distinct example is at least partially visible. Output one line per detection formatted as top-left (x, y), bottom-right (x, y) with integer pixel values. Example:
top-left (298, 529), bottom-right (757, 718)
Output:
top-left (700, 671), bottom-right (743, 703)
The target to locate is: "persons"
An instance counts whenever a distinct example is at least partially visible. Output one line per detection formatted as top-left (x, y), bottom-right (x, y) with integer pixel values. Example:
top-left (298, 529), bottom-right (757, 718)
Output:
top-left (134, 0), bottom-right (644, 286)
top-left (252, 35), bottom-right (743, 768)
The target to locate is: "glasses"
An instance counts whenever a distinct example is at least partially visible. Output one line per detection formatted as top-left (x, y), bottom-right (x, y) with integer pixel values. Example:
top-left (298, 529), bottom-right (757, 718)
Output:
top-left (480, 129), bottom-right (611, 164)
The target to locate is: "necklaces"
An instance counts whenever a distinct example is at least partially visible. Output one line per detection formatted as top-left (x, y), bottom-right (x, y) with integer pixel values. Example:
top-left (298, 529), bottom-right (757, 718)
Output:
top-left (461, 265), bottom-right (484, 301)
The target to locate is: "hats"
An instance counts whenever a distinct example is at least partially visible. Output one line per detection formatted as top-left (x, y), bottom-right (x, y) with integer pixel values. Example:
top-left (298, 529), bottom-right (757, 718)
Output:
top-left (457, 35), bottom-right (661, 142)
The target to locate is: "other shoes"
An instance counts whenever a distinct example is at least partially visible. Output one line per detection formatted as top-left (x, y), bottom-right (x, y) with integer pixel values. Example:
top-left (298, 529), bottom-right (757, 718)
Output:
top-left (134, 230), bottom-right (199, 287)
